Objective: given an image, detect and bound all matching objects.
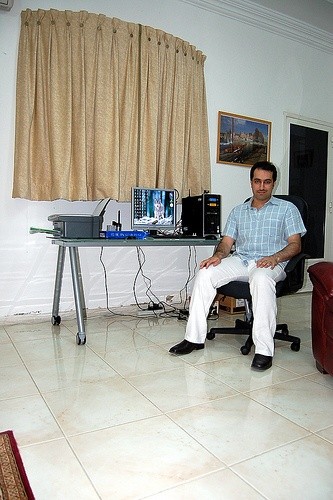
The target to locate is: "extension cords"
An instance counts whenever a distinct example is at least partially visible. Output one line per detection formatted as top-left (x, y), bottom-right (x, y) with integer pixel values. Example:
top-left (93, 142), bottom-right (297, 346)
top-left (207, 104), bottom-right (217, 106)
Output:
top-left (138, 308), bottom-right (165, 316)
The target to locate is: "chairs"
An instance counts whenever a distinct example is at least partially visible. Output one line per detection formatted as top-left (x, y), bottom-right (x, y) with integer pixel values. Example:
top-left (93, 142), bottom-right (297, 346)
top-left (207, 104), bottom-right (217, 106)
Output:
top-left (206, 195), bottom-right (310, 355)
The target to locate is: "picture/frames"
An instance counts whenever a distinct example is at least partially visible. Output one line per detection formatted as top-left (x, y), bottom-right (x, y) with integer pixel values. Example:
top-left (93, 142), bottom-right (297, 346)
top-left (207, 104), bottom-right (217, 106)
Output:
top-left (215, 111), bottom-right (272, 168)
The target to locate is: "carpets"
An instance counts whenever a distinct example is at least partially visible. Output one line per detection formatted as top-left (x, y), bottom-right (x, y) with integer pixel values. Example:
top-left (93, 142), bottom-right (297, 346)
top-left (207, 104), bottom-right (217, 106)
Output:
top-left (0, 430), bottom-right (36, 500)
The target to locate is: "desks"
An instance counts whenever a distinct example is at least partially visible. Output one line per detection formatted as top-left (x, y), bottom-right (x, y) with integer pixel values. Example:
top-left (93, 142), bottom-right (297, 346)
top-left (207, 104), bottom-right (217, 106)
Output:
top-left (45, 236), bottom-right (250, 345)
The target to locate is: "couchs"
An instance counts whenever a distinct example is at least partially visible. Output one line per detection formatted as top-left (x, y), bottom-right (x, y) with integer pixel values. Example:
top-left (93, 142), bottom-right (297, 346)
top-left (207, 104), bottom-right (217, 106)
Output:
top-left (306, 261), bottom-right (333, 378)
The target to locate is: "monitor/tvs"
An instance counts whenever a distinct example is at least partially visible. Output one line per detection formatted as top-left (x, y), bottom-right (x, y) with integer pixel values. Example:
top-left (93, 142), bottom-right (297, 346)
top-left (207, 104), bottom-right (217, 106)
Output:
top-left (129, 187), bottom-right (177, 236)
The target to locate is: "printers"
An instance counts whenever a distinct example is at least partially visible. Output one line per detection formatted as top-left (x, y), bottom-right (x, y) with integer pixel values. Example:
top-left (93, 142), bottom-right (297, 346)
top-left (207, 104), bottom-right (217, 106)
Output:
top-left (47, 196), bottom-right (110, 239)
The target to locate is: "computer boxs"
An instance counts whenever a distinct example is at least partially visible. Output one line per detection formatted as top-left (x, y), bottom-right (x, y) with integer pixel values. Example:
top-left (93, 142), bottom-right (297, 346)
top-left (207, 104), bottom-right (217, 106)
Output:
top-left (181, 194), bottom-right (221, 239)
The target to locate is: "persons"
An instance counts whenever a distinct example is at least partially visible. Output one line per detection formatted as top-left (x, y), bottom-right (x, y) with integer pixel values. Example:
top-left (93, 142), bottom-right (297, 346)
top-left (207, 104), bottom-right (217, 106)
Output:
top-left (168, 161), bottom-right (307, 371)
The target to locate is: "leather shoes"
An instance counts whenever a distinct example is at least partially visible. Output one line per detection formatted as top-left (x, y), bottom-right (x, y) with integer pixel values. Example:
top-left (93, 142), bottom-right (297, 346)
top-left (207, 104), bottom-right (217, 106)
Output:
top-left (251, 354), bottom-right (272, 370)
top-left (169, 340), bottom-right (205, 355)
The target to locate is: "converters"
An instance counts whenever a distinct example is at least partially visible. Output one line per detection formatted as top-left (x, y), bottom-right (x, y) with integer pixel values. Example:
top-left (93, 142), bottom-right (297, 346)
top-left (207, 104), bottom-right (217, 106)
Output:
top-left (180, 308), bottom-right (189, 314)
